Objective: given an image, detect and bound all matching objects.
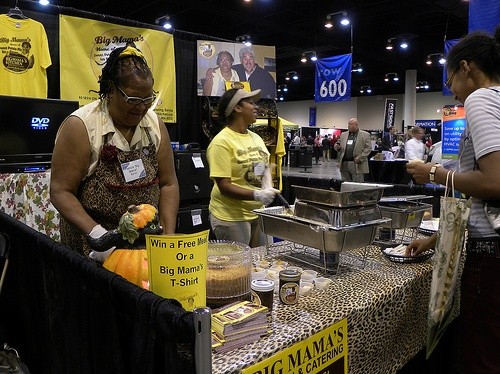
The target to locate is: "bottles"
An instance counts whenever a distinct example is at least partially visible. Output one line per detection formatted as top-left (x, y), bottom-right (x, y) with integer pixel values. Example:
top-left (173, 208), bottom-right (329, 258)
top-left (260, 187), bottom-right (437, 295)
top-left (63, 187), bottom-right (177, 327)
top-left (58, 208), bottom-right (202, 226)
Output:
top-left (337, 139), bottom-right (340, 152)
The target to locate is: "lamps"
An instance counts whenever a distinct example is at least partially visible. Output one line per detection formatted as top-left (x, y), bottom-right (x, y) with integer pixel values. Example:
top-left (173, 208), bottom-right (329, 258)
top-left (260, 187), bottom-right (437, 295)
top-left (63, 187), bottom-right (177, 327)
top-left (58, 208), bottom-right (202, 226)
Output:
top-left (301, 50), bottom-right (318, 63)
top-left (386, 34), bottom-right (409, 51)
top-left (360, 85), bottom-right (371, 94)
top-left (285, 71), bottom-right (298, 80)
top-left (278, 84), bottom-right (288, 92)
top-left (325, 11), bottom-right (349, 29)
top-left (351, 63), bottom-right (363, 72)
top-left (154, 15), bottom-right (172, 30)
top-left (425, 53), bottom-right (446, 65)
top-left (384, 73), bottom-right (399, 82)
top-left (454, 103), bottom-right (464, 110)
top-left (235, 34), bottom-right (252, 48)
top-left (416, 81), bottom-right (429, 89)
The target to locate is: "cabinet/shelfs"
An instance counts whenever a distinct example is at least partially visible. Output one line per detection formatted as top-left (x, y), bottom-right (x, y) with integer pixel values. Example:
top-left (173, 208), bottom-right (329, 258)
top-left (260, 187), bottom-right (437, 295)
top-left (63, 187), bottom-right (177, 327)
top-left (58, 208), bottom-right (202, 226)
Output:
top-left (256, 100), bottom-right (280, 190)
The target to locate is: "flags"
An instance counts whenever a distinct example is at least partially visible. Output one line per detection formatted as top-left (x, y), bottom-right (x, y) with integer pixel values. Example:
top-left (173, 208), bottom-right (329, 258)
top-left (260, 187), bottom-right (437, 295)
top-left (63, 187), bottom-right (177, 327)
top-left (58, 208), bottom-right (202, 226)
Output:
top-left (440, 38), bottom-right (470, 96)
top-left (313, 52), bottom-right (353, 101)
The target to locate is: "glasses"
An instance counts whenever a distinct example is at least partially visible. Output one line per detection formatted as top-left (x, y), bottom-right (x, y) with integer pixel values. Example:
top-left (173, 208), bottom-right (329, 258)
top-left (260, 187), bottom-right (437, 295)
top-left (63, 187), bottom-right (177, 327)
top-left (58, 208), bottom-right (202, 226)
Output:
top-left (114, 83), bottom-right (156, 105)
top-left (444, 59), bottom-right (469, 90)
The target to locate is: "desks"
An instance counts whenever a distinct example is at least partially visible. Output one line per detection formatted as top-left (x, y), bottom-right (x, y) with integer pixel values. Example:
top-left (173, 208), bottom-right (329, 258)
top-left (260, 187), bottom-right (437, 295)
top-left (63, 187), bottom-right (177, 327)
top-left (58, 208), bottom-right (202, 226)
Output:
top-left (364, 160), bottom-right (412, 184)
top-left (212, 227), bottom-right (468, 374)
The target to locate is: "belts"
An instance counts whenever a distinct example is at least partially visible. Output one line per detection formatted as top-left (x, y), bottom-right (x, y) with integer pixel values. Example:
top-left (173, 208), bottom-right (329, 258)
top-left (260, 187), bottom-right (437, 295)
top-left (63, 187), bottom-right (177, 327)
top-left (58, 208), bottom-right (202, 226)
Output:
top-left (466, 238), bottom-right (500, 257)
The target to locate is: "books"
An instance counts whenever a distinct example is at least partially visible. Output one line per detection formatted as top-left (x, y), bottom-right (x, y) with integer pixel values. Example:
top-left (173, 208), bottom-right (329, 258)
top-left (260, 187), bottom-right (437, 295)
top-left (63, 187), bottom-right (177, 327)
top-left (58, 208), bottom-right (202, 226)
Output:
top-left (210, 300), bottom-right (271, 355)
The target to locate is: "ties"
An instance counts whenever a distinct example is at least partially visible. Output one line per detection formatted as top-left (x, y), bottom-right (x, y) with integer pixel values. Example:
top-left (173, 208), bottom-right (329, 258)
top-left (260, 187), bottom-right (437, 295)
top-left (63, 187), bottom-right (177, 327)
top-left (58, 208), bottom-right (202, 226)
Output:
top-left (391, 134), bottom-right (393, 143)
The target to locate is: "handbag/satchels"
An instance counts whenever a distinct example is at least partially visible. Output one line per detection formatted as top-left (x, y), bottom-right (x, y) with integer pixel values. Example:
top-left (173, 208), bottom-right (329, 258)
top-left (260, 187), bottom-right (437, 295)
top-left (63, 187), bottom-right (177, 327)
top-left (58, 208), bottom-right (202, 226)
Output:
top-left (429, 170), bottom-right (470, 328)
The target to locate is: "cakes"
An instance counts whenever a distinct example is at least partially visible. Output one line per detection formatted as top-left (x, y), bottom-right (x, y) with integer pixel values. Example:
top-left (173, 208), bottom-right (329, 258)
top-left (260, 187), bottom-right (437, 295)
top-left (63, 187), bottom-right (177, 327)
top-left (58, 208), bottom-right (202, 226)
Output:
top-left (206, 263), bottom-right (251, 297)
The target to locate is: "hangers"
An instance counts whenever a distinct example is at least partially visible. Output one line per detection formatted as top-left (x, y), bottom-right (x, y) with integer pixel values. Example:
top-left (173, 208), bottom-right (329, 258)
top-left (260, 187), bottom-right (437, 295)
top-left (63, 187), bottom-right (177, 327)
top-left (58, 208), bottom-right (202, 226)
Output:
top-left (6, 0), bottom-right (28, 19)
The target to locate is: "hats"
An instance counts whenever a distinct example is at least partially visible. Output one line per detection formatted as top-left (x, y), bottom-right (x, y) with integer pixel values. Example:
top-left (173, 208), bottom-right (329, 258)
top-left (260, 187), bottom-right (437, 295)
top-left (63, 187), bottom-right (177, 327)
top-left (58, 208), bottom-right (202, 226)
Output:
top-left (225, 89), bottom-right (261, 117)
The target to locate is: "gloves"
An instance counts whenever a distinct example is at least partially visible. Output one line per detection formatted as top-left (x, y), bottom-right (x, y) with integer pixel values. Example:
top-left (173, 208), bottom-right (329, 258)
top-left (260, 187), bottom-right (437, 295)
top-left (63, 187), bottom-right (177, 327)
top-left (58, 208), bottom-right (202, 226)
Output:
top-left (87, 225), bottom-right (116, 261)
top-left (254, 188), bottom-right (279, 206)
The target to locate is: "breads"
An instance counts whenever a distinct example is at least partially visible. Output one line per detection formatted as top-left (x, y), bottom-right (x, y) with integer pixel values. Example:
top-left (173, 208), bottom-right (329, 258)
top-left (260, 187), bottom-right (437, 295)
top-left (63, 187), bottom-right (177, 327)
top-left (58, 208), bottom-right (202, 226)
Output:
top-left (407, 159), bottom-right (425, 174)
top-left (424, 211), bottom-right (432, 220)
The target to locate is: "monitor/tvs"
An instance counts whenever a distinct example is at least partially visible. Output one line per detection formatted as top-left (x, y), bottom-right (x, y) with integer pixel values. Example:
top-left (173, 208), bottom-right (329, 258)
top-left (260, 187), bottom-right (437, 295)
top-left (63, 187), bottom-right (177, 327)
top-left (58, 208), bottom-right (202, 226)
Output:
top-left (0, 95), bottom-right (80, 171)
top-left (192, 37), bottom-right (278, 100)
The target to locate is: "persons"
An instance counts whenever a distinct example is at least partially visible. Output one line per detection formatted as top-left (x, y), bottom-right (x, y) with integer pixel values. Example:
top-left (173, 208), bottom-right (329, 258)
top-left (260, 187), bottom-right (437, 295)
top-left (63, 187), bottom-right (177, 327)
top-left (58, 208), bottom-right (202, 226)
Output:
top-left (203, 51), bottom-right (241, 97)
top-left (334, 119), bottom-right (433, 185)
top-left (46, 39), bottom-right (180, 273)
top-left (404, 29), bottom-right (500, 374)
top-left (203, 89), bottom-right (280, 250)
top-left (206, 47), bottom-right (277, 100)
top-left (281, 127), bottom-right (343, 166)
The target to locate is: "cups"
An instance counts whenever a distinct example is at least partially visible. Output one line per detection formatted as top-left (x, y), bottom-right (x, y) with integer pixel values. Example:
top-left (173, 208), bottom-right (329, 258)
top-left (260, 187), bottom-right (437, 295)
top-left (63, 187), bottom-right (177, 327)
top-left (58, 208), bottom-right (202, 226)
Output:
top-left (246, 260), bottom-right (331, 297)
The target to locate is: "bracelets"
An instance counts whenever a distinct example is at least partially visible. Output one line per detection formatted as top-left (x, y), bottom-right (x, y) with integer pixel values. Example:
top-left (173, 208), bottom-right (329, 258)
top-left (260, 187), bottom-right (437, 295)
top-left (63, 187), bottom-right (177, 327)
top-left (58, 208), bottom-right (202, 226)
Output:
top-left (429, 163), bottom-right (444, 185)
